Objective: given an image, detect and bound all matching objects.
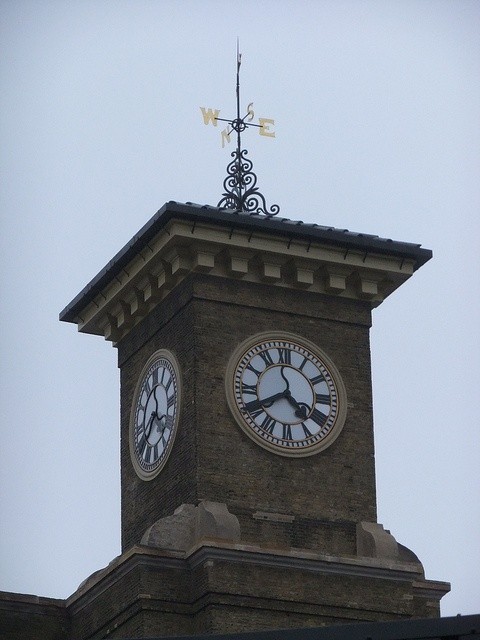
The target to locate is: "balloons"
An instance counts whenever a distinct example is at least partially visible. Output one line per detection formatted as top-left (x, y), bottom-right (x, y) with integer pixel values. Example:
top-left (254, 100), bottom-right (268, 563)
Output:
top-left (224, 329), bottom-right (348, 458)
top-left (128, 347), bottom-right (183, 482)
